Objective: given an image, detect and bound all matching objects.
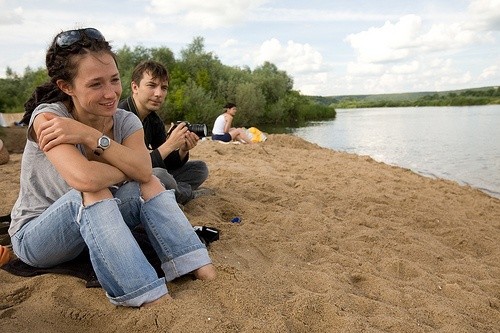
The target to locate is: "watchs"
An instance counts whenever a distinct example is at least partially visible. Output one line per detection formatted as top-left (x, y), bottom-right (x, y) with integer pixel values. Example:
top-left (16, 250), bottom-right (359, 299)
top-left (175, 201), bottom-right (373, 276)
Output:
top-left (94, 134), bottom-right (111, 156)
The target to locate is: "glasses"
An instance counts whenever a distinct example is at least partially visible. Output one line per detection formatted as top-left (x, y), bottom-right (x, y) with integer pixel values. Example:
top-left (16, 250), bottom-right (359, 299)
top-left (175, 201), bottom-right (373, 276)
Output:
top-left (49, 28), bottom-right (106, 66)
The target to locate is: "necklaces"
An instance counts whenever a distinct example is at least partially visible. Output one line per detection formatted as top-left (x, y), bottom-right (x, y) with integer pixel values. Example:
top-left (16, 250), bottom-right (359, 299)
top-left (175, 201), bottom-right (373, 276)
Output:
top-left (103, 117), bottom-right (105, 135)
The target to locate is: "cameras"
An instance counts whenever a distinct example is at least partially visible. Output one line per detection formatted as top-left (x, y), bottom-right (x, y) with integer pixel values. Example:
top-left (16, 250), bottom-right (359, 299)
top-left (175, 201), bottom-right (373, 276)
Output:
top-left (173, 121), bottom-right (207, 139)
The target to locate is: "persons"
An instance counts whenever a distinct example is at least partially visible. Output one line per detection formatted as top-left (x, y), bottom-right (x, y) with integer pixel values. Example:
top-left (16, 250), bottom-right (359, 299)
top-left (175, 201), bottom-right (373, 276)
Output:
top-left (117, 60), bottom-right (216, 210)
top-left (8, 29), bottom-right (217, 308)
top-left (212, 103), bottom-right (253, 144)
